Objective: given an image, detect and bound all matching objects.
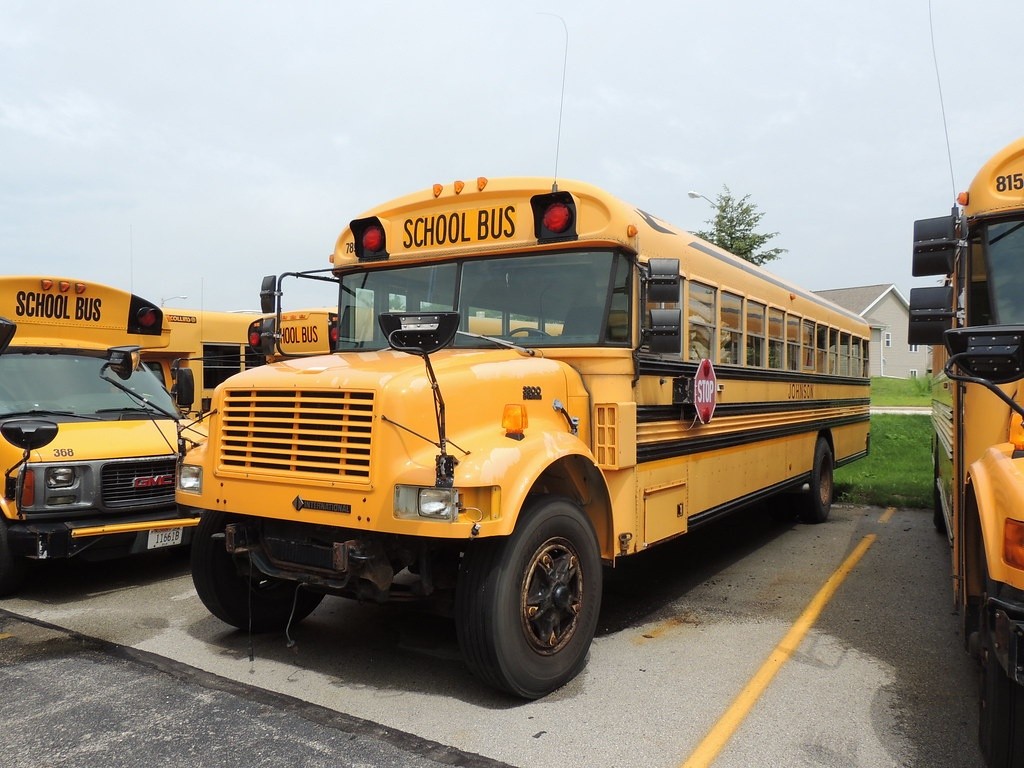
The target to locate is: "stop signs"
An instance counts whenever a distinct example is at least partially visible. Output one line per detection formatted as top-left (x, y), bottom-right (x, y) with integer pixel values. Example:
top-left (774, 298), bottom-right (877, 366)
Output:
top-left (692, 358), bottom-right (720, 425)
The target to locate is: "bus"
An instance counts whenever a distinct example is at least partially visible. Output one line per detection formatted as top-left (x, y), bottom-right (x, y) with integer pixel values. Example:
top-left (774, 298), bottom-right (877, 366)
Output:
top-left (0, 277), bottom-right (569, 599)
top-left (906, 133), bottom-right (1023, 768)
top-left (169, 174), bottom-right (877, 703)
